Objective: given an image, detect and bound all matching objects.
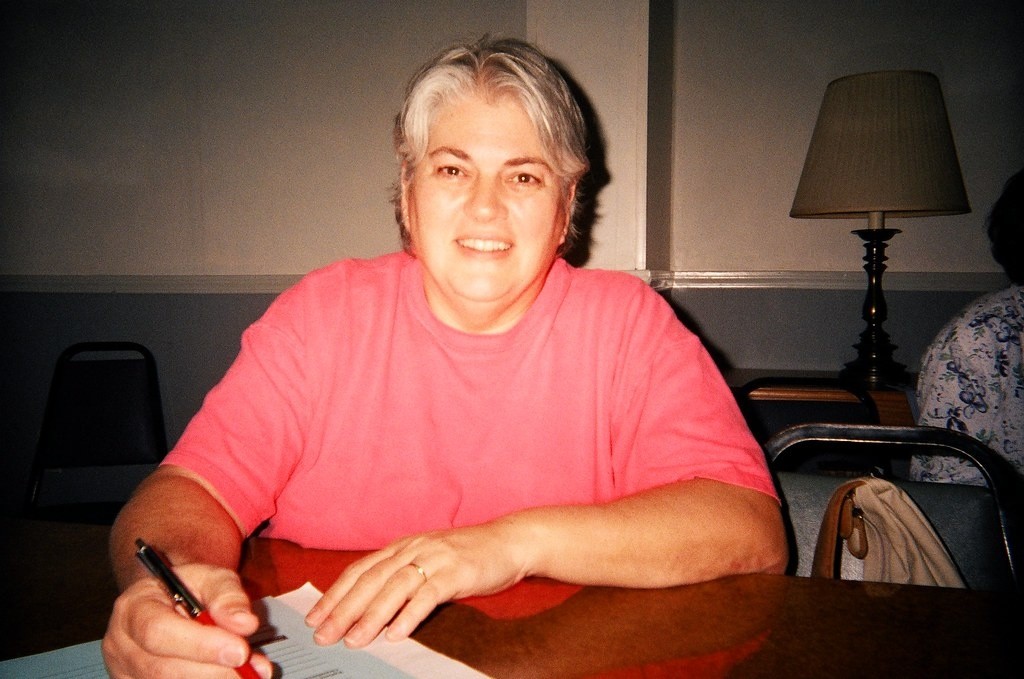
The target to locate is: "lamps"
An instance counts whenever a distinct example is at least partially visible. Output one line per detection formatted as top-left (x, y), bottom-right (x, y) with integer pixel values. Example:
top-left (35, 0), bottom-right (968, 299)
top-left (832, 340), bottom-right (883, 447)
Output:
top-left (789, 71), bottom-right (973, 381)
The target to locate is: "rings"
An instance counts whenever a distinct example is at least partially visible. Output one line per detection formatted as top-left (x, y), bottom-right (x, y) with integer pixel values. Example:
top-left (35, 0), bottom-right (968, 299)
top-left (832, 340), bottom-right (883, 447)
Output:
top-left (407, 563), bottom-right (427, 584)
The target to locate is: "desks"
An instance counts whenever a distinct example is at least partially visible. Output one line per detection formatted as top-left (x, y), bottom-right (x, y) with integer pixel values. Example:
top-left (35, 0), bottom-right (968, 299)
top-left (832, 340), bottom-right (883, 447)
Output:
top-left (726, 367), bottom-right (918, 470)
top-left (0, 518), bottom-right (1024, 679)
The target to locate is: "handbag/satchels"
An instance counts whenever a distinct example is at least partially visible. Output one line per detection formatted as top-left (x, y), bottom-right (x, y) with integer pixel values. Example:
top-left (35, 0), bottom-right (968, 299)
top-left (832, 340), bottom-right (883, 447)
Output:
top-left (811, 476), bottom-right (966, 588)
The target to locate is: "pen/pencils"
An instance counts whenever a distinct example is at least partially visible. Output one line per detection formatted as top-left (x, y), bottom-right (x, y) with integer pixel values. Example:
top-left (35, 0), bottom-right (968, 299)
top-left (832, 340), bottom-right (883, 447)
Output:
top-left (132, 536), bottom-right (264, 679)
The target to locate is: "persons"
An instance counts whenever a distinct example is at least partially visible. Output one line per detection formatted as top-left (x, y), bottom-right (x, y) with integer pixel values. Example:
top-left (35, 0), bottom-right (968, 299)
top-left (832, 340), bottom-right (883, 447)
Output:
top-left (910, 171), bottom-right (1024, 488)
top-left (100, 36), bottom-right (787, 679)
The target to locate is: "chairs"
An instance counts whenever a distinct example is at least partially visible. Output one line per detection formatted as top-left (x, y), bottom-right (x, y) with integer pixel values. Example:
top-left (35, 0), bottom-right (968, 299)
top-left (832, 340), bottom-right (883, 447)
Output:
top-left (30, 342), bottom-right (168, 525)
top-left (763, 423), bottom-right (1024, 589)
